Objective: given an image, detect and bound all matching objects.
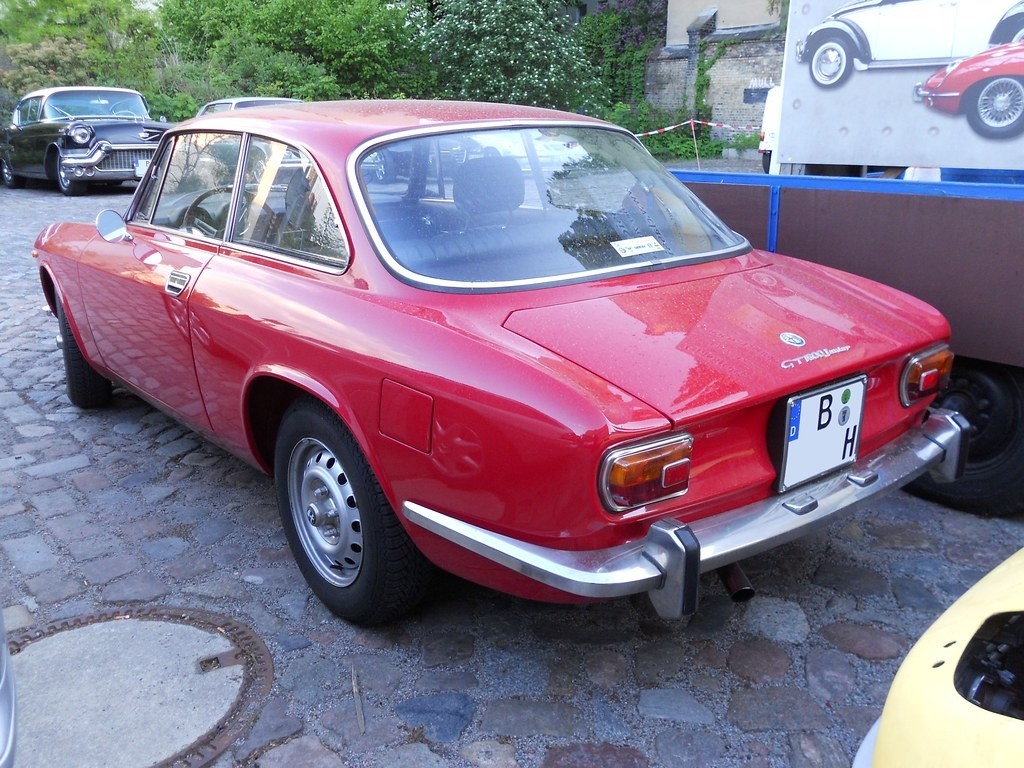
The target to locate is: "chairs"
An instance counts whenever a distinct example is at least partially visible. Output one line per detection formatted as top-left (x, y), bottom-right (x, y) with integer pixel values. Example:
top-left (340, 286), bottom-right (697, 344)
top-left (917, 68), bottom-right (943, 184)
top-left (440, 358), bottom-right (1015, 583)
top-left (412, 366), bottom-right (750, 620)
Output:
top-left (437, 155), bottom-right (526, 238)
top-left (286, 164), bottom-right (347, 259)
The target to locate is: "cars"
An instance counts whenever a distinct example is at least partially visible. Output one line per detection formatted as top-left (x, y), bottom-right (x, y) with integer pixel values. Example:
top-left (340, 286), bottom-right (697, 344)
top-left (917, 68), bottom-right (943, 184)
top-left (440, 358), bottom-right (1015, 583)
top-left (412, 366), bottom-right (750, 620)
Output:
top-left (796, 0), bottom-right (1024, 88)
top-left (912, 37), bottom-right (1024, 138)
top-left (852, 540), bottom-right (1024, 766)
top-left (757, 85), bottom-right (782, 174)
top-left (0, 85), bottom-right (182, 197)
top-left (200, 97), bottom-right (304, 115)
top-left (33, 98), bottom-right (971, 630)
